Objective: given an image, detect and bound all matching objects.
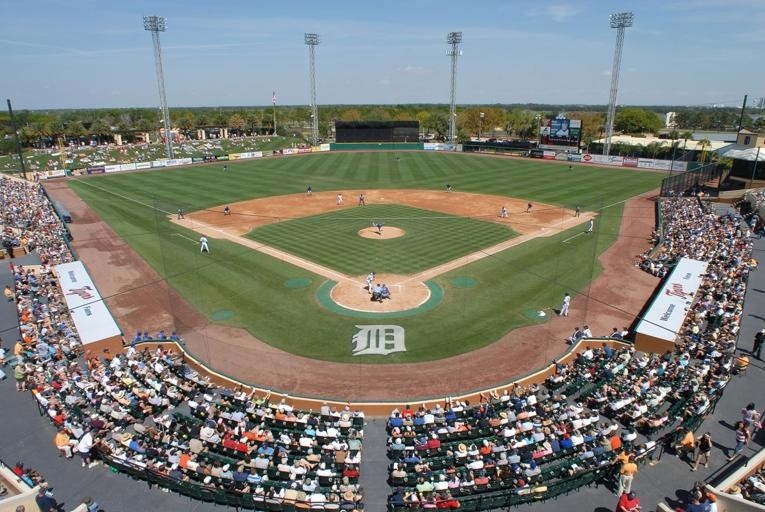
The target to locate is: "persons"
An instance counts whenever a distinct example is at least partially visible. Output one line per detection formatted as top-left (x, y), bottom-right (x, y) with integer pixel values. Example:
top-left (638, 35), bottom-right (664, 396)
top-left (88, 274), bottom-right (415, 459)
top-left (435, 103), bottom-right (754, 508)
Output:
top-left (447, 182), bottom-right (452, 193)
top-left (336, 195), bottom-right (343, 204)
top-left (1, 176), bottom-right (79, 339)
top-left (372, 283), bottom-right (384, 303)
top-left (224, 207), bottom-right (232, 215)
top-left (188, 360), bottom-right (765, 511)
top-left (366, 271), bottom-right (377, 294)
top-left (380, 284), bottom-right (391, 300)
top-left (560, 292), bottom-right (570, 317)
top-left (372, 221), bottom-right (387, 231)
top-left (307, 187), bottom-right (312, 193)
top-left (567, 183), bottom-right (765, 359)
top-left (527, 200), bottom-right (534, 213)
top-left (556, 121), bottom-right (570, 137)
top-left (502, 206), bottom-right (509, 219)
top-left (0, 331), bottom-right (192, 512)
top-left (358, 194), bottom-right (366, 207)
top-left (0, 131), bottom-right (310, 179)
top-left (198, 234), bottom-right (209, 254)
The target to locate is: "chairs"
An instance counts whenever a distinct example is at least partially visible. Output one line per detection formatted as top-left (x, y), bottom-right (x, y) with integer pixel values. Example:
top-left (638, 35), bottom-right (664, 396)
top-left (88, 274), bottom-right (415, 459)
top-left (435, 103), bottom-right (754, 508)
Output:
top-left (35, 353), bottom-right (363, 511)
top-left (389, 347), bottom-right (736, 512)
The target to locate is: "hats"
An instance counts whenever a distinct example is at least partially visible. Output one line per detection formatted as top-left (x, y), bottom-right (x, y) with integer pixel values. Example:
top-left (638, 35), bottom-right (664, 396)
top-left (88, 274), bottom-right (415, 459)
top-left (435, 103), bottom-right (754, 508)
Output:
top-left (396, 391), bottom-right (636, 500)
top-left (115, 398), bottom-right (355, 500)
top-left (706, 492), bottom-right (717, 501)
top-left (729, 484), bottom-right (742, 495)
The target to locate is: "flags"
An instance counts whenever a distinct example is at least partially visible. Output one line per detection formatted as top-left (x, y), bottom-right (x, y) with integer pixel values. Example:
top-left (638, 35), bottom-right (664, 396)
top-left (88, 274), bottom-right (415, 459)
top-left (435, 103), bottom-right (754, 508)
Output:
top-left (272, 92), bottom-right (277, 104)
